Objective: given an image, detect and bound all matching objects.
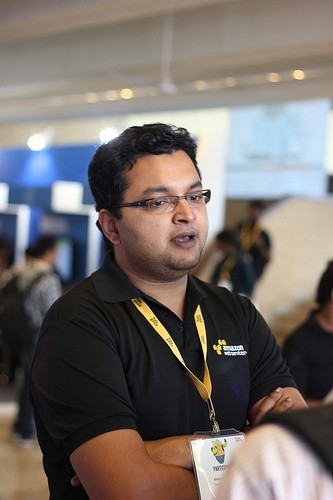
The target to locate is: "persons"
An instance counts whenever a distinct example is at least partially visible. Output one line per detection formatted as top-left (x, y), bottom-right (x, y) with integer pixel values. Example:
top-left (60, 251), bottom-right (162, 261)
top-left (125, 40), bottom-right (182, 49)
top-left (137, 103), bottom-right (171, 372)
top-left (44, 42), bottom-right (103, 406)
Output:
top-left (212, 403), bottom-right (333, 500)
top-left (0, 197), bottom-right (333, 447)
top-left (29, 124), bottom-right (309, 500)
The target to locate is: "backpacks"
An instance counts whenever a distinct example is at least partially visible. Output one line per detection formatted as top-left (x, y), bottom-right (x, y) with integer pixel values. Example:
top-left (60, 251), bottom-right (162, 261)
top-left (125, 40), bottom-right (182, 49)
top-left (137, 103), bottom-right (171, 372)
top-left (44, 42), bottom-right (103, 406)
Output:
top-left (0, 272), bottom-right (50, 362)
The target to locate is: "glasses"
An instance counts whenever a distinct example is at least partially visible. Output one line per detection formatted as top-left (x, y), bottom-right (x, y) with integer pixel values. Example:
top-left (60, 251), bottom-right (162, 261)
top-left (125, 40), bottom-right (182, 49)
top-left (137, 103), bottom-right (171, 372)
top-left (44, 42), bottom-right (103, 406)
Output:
top-left (116, 189), bottom-right (213, 216)
